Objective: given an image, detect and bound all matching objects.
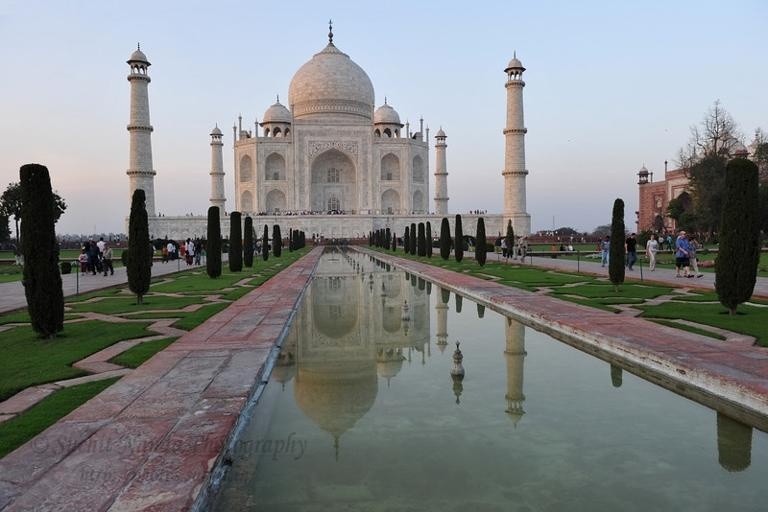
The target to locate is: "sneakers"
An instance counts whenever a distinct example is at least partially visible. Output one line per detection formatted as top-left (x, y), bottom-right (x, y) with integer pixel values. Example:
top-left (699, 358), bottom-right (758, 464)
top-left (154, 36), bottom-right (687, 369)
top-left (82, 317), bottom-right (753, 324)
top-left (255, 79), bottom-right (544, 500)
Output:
top-left (601, 264), bottom-right (605, 267)
top-left (675, 274), bottom-right (681, 278)
top-left (683, 273), bottom-right (695, 278)
top-left (628, 266), bottom-right (633, 271)
top-left (650, 268), bottom-right (655, 271)
top-left (696, 274), bottom-right (703, 278)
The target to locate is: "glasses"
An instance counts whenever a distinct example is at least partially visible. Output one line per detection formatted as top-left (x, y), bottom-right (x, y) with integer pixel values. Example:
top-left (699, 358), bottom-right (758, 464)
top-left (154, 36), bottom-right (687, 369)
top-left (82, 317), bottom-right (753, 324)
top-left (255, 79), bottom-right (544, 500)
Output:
top-left (683, 234), bottom-right (686, 235)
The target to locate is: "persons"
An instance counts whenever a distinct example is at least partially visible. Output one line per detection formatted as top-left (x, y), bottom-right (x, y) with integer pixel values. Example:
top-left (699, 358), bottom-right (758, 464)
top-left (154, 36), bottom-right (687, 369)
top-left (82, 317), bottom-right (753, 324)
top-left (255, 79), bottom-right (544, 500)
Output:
top-left (501, 234), bottom-right (574, 261)
top-left (312, 233), bottom-right (320, 246)
top-left (601, 231), bottom-right (702, 278)
top-left (162, 237), bottom-right (202, 266)
top-left (78, 237), bottom-right (114, 276)
